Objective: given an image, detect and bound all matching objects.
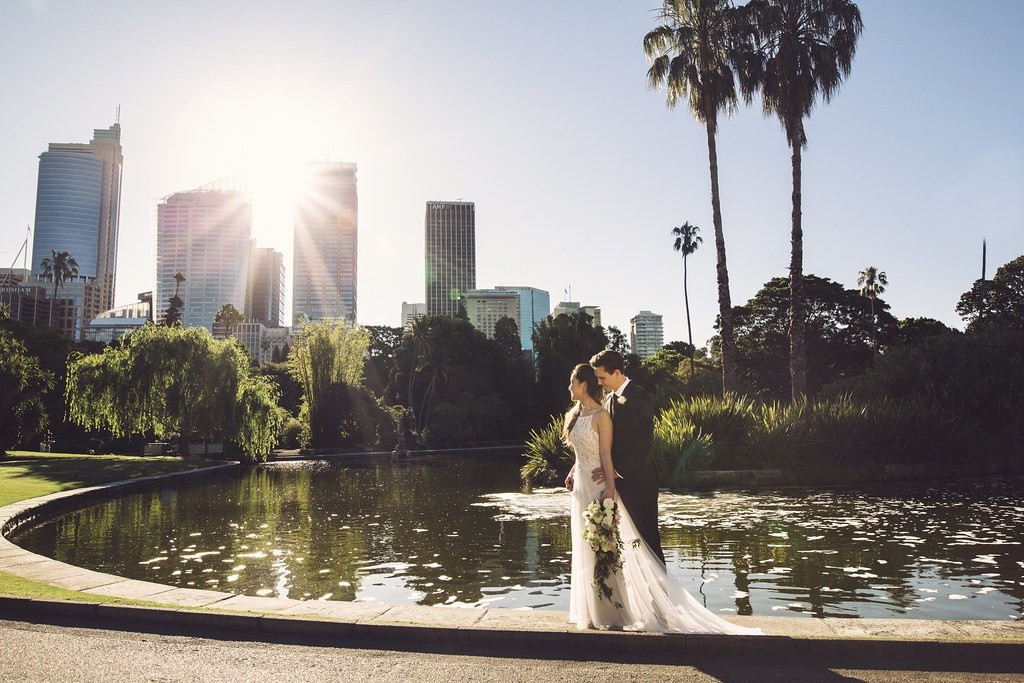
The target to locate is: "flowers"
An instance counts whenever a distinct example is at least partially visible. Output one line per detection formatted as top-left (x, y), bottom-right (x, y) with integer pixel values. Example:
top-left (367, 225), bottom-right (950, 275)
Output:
top-left (582, 498), bottom-right (642, 619)
top-left (617, 396), bottom-right (627, 405)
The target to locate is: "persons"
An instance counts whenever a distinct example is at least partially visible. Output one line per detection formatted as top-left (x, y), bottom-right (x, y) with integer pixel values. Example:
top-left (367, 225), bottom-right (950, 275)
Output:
top-left (589, 350), bottom-right (669, 631)
top-left (560, 364), bottom-right (763, 635)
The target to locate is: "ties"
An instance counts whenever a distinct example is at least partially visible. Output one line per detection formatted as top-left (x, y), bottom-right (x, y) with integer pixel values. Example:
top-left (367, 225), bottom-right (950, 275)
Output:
top-left (613, 394), bottom-right (617, 402)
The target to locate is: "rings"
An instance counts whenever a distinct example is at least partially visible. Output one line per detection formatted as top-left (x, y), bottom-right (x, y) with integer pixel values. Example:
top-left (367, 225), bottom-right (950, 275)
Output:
top-left (600, 474), bottom-right (603, 478)
